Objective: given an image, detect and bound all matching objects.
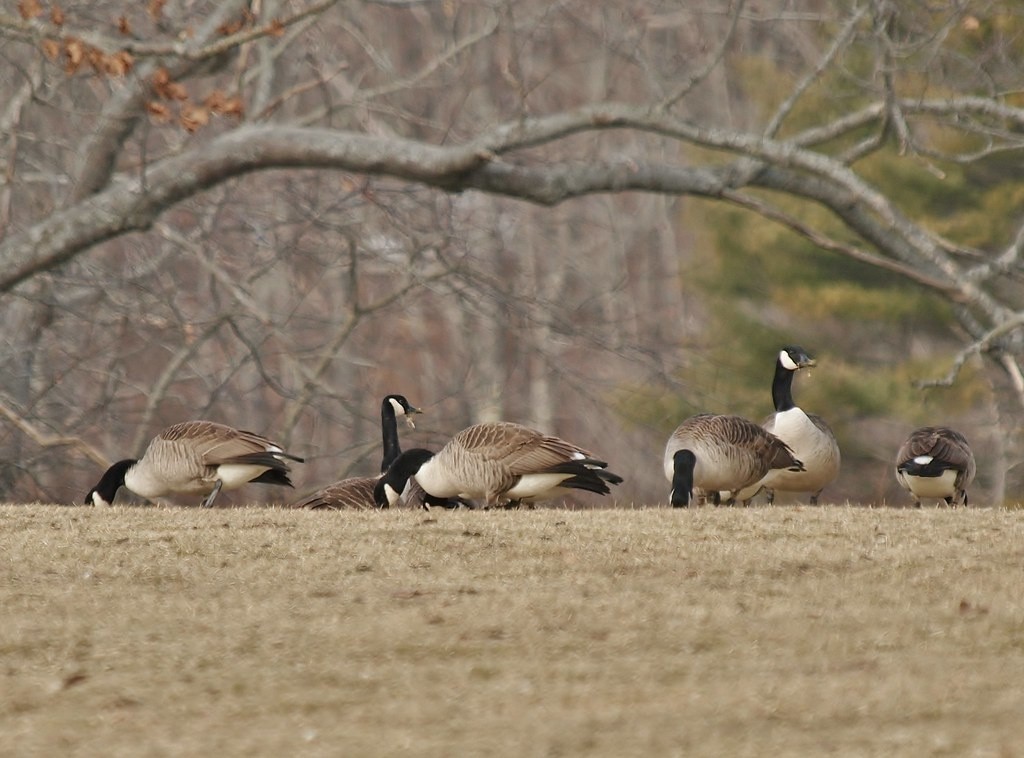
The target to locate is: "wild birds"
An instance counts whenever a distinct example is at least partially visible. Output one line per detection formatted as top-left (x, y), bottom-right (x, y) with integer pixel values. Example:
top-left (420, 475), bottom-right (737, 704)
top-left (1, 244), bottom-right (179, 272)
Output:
top-left (662, 413), bottom-right (808, 509)
top-left (752, 346), bottom-right (841, 505)
top-left (286, 394), bottom-right (423, 508)
top-left (373, 421), bottom-right (624, 508)
top-left (84, 421), bottom-right (304, 508)
top-left (893, 426), bottom-right (977, 509)
